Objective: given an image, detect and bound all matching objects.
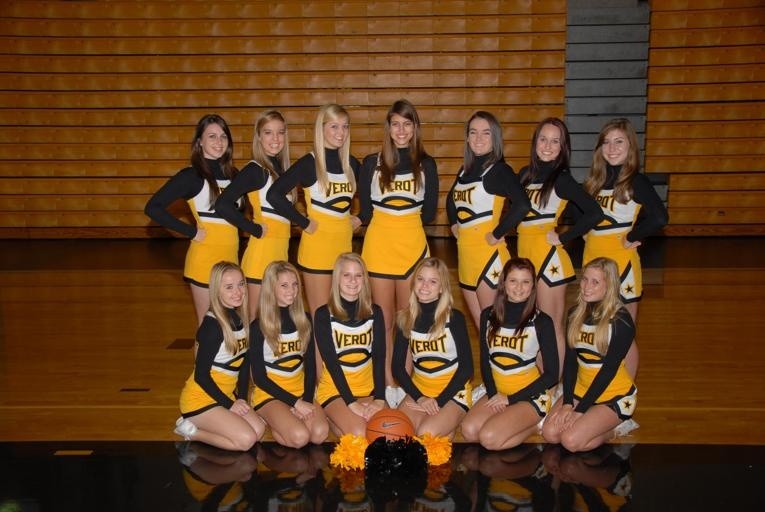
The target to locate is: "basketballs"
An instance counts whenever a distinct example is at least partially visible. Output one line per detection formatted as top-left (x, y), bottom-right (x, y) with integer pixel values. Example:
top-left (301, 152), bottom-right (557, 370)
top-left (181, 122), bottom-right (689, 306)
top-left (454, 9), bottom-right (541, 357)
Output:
top-left (365, 409), bottom-right (414, 444)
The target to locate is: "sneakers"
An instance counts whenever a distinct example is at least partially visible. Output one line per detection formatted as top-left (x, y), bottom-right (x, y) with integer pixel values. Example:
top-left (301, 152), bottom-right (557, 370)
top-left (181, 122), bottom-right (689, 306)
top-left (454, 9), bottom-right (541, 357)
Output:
top-left (385, 385), bottom-right (397, 409)
top-left (172, 419), bottom-right (196, 441)
top-left (613, 418), bottom-right (641, 437)
top-left (471, 382), bottom-right (486, 402)
top-left (397, 386), bottom-right (407, 406)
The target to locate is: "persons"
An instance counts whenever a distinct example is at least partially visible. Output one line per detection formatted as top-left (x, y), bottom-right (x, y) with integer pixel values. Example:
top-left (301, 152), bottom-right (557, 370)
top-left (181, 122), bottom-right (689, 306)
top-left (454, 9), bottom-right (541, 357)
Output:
top-left (214, 111), bottom-right (297, 387)
top-left (540, 255), bottom-right (639, 451)
top-left (570, 123), bottom-right (671, 383)
top-left (360, 99), bottom-right (440, 409)
top-left (389, 259), bottom-right (474, 447)
top-left (144, 115), bottom-right (246, 364)
top-left (265, 104), bottom-right (374, 390)
top-left (171, 443), bottom-right (635, 512)
top-left (446, 111), bottom-right (531, 338)
top-left (314, 253), bottom-right (392, 444)
top-left (459, 258), bottom-right (554, 450)
top-left (172, 261), bottom-right (265, 451)
top-left (505, 117), bottom-right (604, 406)
top-left (245, 261), bottom-right (331, 450)
